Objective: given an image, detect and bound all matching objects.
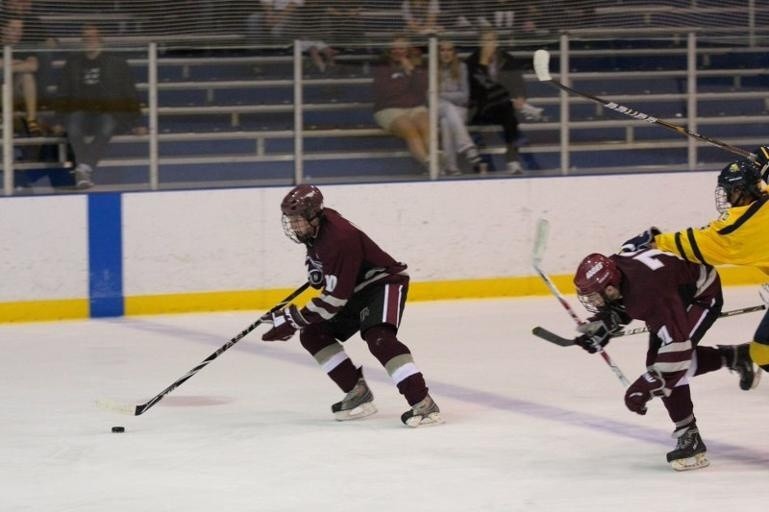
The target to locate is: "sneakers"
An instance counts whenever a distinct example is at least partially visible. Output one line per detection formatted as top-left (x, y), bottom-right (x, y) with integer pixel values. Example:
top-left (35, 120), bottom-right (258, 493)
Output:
top-left (519, 103), bottom-right (545, 121)
top-left (506, 161), bottom-right (524, 175)
top-left (69, 163), bottom-right (95, 189)
top-left (423, 151), bottom-right (485, 177)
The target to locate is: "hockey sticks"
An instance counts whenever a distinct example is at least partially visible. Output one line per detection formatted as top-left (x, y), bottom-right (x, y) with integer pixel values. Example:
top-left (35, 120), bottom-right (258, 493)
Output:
top-left (97, 281), bottom-right (309, 416)
top-left (531, 220), bottom-right (630, 389)
top-left (533, 305), bottom-right (765, 346)
top-left (533, 49), bottom-right (758, 163)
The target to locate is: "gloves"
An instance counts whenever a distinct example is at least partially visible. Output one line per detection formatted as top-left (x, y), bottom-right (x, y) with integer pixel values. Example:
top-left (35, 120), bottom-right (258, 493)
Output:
top-left (752, 146), bottom-right (769, 185)
top-left (625, 368), bottom-right (673, 415)
top-left (575, 311), bottom-right (624, 353)
top-left (260, 304), bottom-right (312, 341)
top-left (618, 226), bottom-right (662, 255)
top-left (305, 256), bottom-right (326, 289)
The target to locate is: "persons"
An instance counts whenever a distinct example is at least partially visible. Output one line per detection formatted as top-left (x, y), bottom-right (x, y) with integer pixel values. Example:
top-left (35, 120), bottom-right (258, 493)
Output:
top-left (620, 145), bottom-right (769, 373)
top-left (372, 28), bottom-right (544, 179)
top-left (1, 14), bottom-right (46, 142)
top-left (53, 23), bottom-right (143, 189)
top-left (1, 1), bottom-right (553, 82)
top-left (259, 184), bottom-right (439, 426)
top-left (573, 248), bottom-right (754, 461)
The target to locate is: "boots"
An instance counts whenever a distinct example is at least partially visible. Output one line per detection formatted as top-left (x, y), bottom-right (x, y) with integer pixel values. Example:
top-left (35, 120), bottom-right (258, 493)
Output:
top-left (667, 423), bottom-right (706, 462)
top-left (401, 392), bottom-right (439, 424)
top-left (331, 365), bottom-right (374, 413)
top-left (717, 344), bottom-right (754, 390)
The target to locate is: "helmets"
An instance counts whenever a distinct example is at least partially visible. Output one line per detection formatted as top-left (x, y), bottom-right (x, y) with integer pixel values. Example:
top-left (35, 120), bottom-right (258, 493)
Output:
top-left (573, 253), bottom-right (622, 314)
top-left (715, 160), bottom-right (762, 215)
top-left (281, 183), bottom-right (323, 243)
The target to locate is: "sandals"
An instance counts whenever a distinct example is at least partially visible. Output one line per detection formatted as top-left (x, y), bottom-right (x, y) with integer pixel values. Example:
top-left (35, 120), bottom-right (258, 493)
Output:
top-left (24, 119), bottom-right (40, 137)
